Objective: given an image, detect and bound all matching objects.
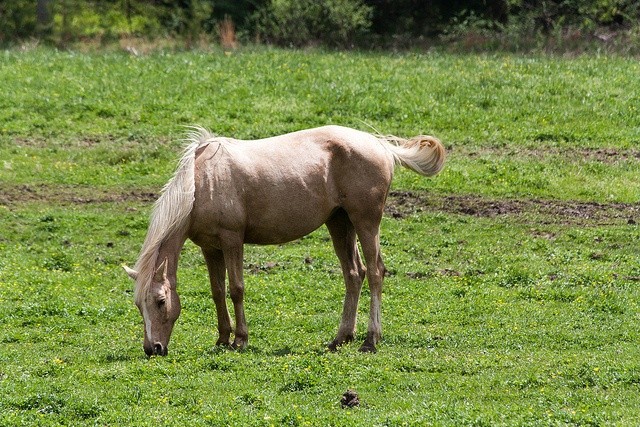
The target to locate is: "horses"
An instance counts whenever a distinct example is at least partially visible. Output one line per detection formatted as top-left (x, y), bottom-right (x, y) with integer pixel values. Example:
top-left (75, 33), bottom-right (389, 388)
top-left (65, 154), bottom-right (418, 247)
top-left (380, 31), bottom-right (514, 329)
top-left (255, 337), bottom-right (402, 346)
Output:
top-left (119, 123), bottom-right (447, 356)
